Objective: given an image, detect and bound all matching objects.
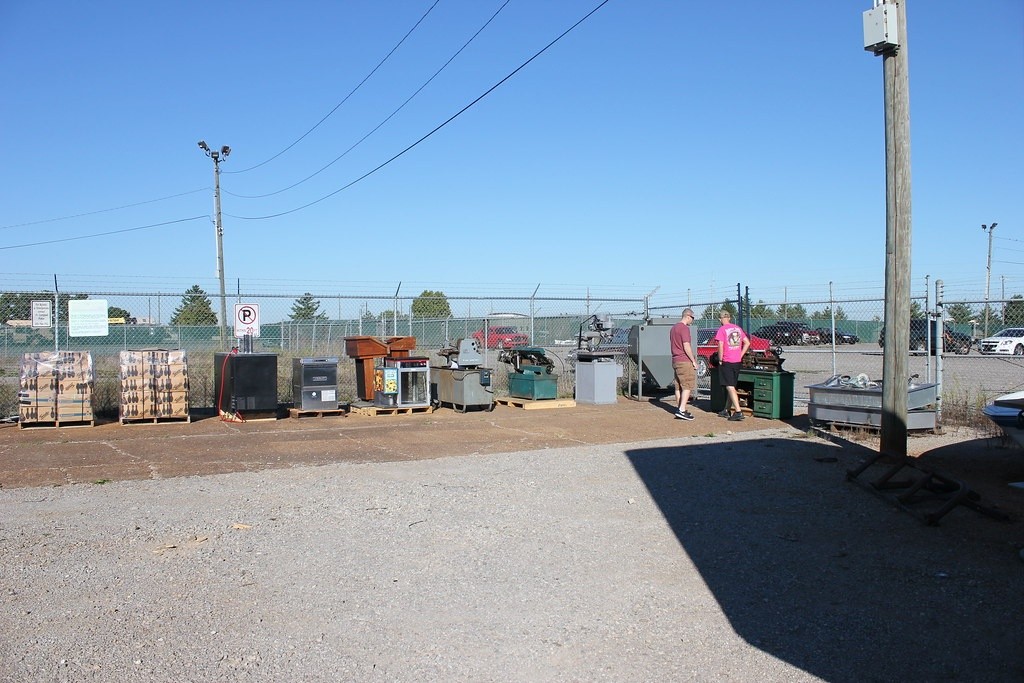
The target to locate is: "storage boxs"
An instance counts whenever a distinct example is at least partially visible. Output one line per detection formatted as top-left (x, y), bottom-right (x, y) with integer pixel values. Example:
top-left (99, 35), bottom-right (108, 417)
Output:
top-left (119, 350), bottom-right (189, 419)
top-left (292, 357), bottom-right (340, 411)
top-left (19, 351), bottom-right (93, 421)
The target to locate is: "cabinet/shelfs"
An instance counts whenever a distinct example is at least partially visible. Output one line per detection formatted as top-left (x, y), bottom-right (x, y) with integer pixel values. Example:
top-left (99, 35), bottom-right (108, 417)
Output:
top-left (709, 368), bottom-right (796, 419)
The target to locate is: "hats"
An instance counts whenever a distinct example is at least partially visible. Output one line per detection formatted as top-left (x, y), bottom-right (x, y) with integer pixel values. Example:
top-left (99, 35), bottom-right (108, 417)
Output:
top-left (718, 310), bottom-right (730, 318)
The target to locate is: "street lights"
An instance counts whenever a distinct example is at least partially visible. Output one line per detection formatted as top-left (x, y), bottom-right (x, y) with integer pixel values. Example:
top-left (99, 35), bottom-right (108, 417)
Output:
top-left (982, 223), bottom-right (998, 338)
top-left (198, 141), bottom-right (231, 353)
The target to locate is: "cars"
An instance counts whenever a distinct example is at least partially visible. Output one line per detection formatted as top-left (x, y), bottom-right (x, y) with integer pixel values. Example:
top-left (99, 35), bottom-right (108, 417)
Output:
top-left (262, 337), bottom-right (288, 348)
top-left (612, 326), bottom-right (632, 345)
top-left (697, 328), bottom-right (772, 378)
top-left (776, 321), bottom-right (821, 346)
top-left (977, 328), bottom-right (1024, 356)
top-left (816, 327), bottom-right (860, 344)
top-left (471, 326), bottom-right (529, 349)
top-left (751, 326), bottom-right (795, 346)
top-left (878, 318), bottom-right (972, 356)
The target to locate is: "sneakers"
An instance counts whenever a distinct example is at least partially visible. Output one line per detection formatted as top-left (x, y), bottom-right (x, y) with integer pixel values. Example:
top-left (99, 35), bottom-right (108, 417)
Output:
top-left (728, 410), bottom-right (744, 420)
top-left (718, 409), bottom-right (731, 418)
top-left (675, 407), bottom-right (696, 421)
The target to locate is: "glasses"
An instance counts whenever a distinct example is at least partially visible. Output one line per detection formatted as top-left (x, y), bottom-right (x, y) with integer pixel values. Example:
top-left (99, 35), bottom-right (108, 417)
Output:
top-left (687, 315), bottom-right (694, 321)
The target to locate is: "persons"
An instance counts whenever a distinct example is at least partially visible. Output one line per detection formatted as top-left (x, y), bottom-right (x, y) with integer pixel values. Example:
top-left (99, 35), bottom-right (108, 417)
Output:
top-left (714, 310), bottom-right (750, 421)
top-left (670, 308), bottom-right (699, 420)
